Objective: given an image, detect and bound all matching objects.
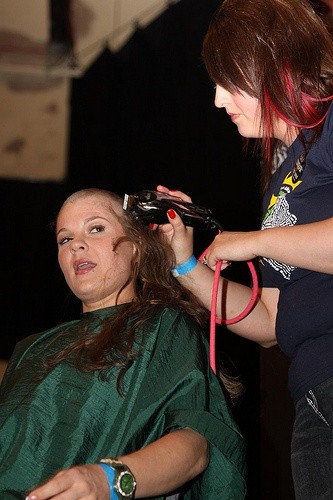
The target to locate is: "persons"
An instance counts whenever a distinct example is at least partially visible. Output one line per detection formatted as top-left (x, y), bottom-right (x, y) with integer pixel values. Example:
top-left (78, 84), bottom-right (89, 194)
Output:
top-left (0, 188), bottom-right (248, 500)
top-left (151, 1), bottom-right (333, 500)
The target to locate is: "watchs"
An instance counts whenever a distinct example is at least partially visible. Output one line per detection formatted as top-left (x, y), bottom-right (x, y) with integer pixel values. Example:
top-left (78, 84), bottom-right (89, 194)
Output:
top-left (100, 459), bottom-right (136, 500)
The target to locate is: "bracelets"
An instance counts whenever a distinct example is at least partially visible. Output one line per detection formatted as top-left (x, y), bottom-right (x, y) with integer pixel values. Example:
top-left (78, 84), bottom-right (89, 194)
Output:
top-left (98, 463), bottom-right (119, 500)
top-left (171, 255), bottom-right (197, 277)
